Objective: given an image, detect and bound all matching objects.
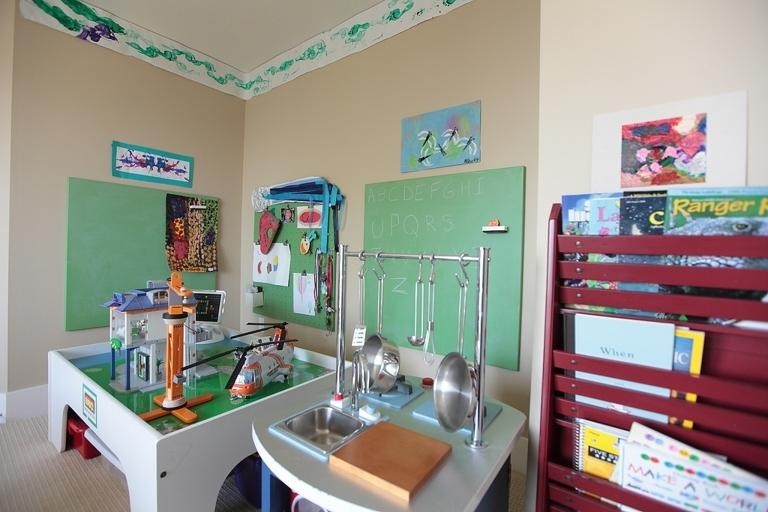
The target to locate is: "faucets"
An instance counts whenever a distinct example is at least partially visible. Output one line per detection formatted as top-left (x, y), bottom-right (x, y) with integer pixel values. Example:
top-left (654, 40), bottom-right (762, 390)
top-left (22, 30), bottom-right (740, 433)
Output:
top-left (351, 349), bottom-right (370, 412)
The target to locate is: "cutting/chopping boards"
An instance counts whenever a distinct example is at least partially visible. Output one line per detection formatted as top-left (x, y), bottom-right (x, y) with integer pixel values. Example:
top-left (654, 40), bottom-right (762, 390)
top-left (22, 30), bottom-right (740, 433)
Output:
top-left (331, 420), bottom-right (452, 503)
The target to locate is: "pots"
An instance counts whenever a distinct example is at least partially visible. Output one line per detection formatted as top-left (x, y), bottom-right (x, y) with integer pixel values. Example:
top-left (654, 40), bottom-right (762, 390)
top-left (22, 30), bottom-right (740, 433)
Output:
top-left (429, 281), bottom-right (479, 431)
top-left (359, 277), bottom-right (400, 396)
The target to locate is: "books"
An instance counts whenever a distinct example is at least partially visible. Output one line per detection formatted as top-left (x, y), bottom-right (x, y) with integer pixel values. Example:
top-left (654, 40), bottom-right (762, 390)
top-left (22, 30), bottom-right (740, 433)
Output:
top-left (571, 418), bottom-right (767, 512)
top-left (563, 185), bottom-right (767, 331)
top-left (562, 314), bottom-right (705, 428)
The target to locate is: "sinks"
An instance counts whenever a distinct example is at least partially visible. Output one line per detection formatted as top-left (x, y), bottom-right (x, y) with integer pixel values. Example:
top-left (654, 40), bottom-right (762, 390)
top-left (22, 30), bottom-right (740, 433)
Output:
top-left (276, 402), bottom-right (373, 454)
